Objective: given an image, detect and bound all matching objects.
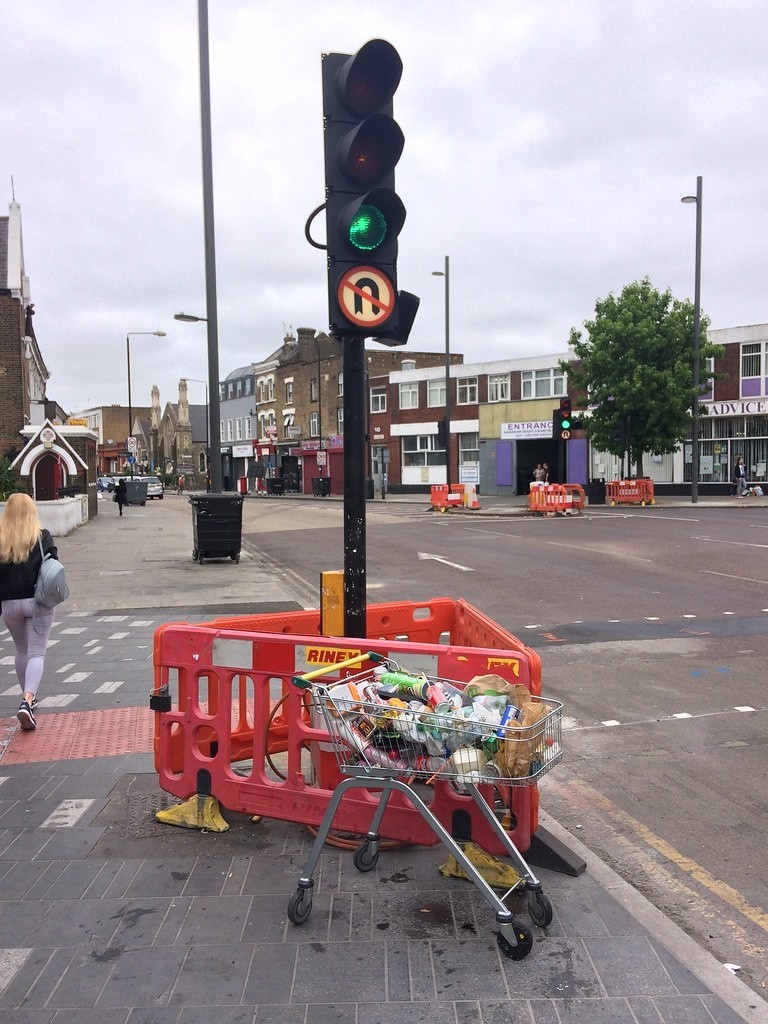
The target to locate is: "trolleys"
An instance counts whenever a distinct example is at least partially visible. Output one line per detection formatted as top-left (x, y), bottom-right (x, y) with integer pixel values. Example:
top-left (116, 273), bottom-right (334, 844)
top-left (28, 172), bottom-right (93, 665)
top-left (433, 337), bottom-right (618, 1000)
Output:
top-left (287, 650), bottom-right (564, 961)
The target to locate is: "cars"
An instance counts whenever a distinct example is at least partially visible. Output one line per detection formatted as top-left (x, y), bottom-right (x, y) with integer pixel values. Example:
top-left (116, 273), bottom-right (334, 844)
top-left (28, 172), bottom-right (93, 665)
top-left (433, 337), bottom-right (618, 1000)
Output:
top-left (97, 476), bottom-right (165, 499)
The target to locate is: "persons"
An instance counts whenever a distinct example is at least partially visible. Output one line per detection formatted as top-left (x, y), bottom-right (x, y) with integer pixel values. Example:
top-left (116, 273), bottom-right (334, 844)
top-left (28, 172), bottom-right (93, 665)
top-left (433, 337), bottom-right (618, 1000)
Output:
top-left (533, 463), bottom-right (551, 483)
top-left (178, 475), bottom-right (185, 495)
top-left (115, 479), bottom-right (127, 516)
top-left (0, 494), bottom-right (59, 729)
top-left (736, 458), bottom-right (747, 498)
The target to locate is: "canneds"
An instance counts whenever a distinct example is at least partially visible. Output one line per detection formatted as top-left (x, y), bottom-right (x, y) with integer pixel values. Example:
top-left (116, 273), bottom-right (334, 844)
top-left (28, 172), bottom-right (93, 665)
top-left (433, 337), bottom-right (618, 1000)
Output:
top-left (481, 704), bottom-right (520, 756)
top-left (337, 674), bottom-right (449, 776)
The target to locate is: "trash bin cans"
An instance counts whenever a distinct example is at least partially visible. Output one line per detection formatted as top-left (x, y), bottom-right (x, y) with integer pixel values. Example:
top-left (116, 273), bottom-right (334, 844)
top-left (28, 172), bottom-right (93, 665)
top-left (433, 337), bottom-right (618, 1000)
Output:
top-left (188, 494), bottom-right (245, 566)
top-left (311, 477), bottom-right (331, 497)
top-left (126, 482), bottom-right (148, 506)
top-left (265, 478), bottom-right (285, 496)
top-left (588, 478), bottom-right (606, 504)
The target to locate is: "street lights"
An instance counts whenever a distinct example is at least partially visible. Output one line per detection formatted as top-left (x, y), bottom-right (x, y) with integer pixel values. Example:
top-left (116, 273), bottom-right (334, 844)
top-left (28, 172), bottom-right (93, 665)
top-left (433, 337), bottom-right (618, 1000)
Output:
top-left (681, 176), bottom-right (703, 503)
top-left (180, 377), bottom-right (223, 493)
top-left (286, 340), bottom-right (323, 479)
top-left (432, 255), bottom-right (451, 485)
top-left (126, 330), bottom-right (167, 482)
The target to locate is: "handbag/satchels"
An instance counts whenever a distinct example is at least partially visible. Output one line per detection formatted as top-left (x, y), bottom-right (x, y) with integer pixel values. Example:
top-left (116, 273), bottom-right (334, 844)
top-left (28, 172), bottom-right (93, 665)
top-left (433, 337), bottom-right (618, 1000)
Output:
top-left (112, 492), bottom-right (117, 502)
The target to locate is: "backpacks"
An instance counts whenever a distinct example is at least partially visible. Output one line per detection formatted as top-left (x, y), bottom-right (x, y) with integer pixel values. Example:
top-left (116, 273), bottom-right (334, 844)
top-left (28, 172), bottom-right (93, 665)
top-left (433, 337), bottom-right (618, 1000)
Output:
top-left (31, 554), bottom-right (70, 607)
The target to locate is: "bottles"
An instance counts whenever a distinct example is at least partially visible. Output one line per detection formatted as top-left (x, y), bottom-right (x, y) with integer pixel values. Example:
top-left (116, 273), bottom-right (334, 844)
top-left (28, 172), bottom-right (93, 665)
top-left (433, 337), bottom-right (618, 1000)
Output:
top-left (337, 673), bottom-right (559, 783)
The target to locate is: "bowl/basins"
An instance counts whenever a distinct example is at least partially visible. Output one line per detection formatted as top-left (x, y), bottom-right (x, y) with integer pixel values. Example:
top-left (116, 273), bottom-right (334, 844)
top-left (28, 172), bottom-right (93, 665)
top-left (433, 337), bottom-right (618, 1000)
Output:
top-left (453, 748), bottom-right (488, 775)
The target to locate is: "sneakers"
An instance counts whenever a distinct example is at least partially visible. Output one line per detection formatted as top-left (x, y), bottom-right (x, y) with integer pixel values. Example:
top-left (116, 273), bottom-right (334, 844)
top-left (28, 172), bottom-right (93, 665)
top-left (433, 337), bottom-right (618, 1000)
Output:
top-left (17, 697), bottom-right (39, 729)
top-left (22, 693), bottom-right (37, 708)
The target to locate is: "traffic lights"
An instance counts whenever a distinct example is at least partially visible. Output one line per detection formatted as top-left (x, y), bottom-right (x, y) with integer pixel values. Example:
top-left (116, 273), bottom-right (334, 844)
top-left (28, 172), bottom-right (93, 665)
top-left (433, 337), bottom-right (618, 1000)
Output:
top-left (560, 397), bottom-right (572, 430)
top-left (322, 39), bottom-right (407, 265)
